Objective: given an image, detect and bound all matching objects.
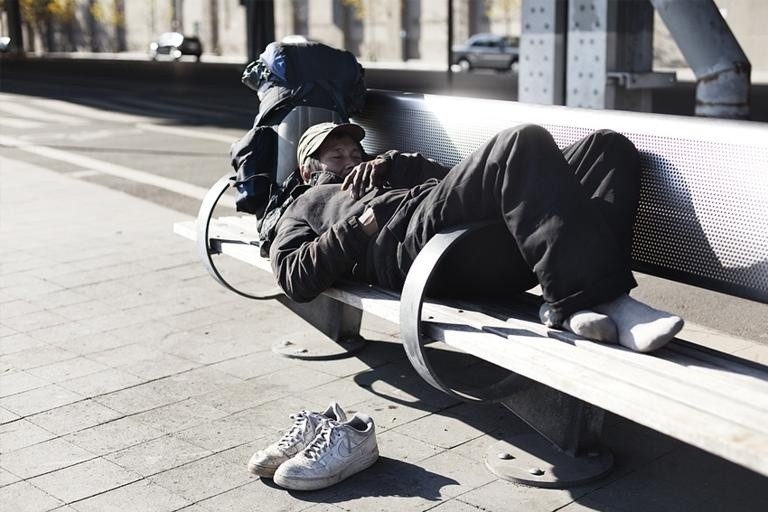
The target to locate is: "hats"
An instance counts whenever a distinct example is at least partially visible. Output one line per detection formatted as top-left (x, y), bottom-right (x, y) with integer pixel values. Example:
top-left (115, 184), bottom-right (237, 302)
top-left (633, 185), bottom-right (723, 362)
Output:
top-left (295, 120), bottom-right (366, 165)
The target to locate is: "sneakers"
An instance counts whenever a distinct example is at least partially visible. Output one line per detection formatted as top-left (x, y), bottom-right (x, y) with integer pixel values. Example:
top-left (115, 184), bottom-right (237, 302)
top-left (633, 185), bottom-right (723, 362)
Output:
top-left (247, 399), bottom-right (381, 494)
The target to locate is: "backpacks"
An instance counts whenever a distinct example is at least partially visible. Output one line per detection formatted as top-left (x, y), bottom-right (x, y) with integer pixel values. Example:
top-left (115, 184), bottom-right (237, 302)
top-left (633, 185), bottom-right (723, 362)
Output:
top-left (254, 39), bottom-right (370, 127)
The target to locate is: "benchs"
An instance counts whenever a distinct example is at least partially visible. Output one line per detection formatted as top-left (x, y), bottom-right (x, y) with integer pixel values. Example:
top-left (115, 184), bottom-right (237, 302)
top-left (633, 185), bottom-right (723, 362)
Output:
top-left (197, 89), bottom-right (768, 482)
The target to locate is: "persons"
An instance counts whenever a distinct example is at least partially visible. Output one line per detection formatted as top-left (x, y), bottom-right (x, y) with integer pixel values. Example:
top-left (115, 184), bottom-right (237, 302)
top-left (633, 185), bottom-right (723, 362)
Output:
top-left (269, 122), bottom-right (684, 353)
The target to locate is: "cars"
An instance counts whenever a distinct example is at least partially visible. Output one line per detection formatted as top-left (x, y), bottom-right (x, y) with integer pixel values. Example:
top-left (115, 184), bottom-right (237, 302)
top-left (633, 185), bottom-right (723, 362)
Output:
top-left (449, 26), bottom-right (521, 75)
top-left (145, 30), bottom-right (205, 62)
top-left (0, 35), bottom-right (10, 51)
